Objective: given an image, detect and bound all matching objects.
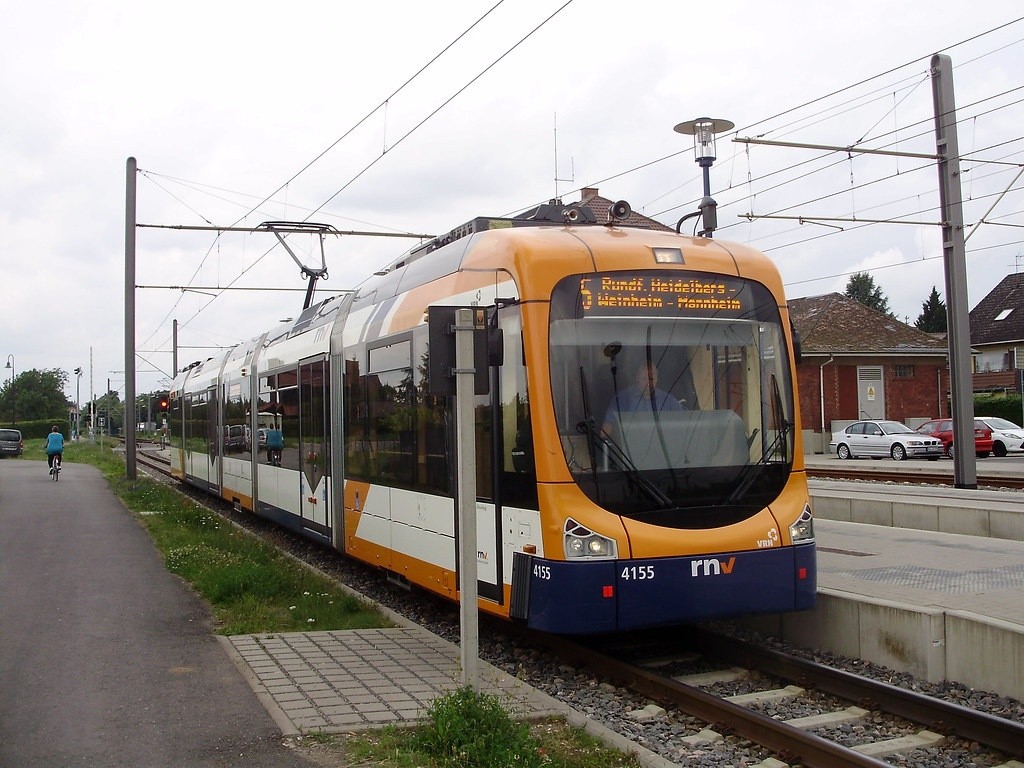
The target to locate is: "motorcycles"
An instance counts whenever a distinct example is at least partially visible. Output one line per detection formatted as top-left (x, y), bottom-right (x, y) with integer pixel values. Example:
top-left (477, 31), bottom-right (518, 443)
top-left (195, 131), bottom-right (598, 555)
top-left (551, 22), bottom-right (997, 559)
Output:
top-left (267, 446), bottom-right (282, 467)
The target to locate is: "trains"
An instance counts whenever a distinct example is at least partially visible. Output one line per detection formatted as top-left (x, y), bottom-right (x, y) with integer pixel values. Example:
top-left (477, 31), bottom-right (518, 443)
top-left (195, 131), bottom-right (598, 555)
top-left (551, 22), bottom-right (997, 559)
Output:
top-left (171, 192), bottom-right (820, 633)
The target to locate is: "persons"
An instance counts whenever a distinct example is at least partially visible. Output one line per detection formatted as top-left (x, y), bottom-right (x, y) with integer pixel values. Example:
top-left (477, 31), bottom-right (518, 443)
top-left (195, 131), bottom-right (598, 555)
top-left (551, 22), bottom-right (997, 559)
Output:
top-left (44, 425), bottom-right (64, 474)
top-left (601, 357), bottom-right (686, 436)
top-left (72, 429), bottom-right (75, 439)
top-left (265, 423), bottom-right (284, 466)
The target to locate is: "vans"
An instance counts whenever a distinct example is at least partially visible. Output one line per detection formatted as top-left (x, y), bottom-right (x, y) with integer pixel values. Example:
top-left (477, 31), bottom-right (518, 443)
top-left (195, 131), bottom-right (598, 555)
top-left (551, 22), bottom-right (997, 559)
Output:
top-left (0, 428), bottom-right (23, 457)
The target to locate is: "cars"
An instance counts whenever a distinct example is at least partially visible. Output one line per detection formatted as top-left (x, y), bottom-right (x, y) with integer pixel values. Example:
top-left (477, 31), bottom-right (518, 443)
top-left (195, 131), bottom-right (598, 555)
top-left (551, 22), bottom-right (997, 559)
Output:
top-left (974, 416), bottom-right (1023, 457)
top-left (829, 419), bottom-right (945, 461)
top-left (210, 424), bottom-right (284, 450)
top-left (915, 417), bottom-right (994, 459)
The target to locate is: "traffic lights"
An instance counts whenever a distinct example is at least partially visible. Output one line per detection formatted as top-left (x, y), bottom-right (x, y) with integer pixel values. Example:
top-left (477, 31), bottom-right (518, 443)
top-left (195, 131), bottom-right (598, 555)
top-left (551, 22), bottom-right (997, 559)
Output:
top-left (160, 398), bottom-right (169, 408)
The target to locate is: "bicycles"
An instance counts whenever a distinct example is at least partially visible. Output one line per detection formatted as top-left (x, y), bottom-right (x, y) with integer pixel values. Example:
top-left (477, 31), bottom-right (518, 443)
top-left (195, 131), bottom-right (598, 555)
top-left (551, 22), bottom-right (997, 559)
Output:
top-left (45, 444), bottom-right (62, 481)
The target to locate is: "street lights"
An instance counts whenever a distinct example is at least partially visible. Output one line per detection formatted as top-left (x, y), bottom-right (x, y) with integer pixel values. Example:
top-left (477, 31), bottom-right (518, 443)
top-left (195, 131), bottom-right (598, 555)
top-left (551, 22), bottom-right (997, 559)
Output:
top-left (73, 367), bottom-right (84, 439)
top-left (669, 118), bottom-right (737, 238)
top-left (4, 355), bottom-right (16, 429)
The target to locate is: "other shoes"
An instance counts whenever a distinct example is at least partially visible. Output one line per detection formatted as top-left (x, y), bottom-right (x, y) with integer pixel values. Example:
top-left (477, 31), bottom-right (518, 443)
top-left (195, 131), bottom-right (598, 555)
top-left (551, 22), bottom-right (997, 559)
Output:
top-left (56, 465), bottom-right (62, 473)
top-left (48, 467), bottom-right (54, 476)
